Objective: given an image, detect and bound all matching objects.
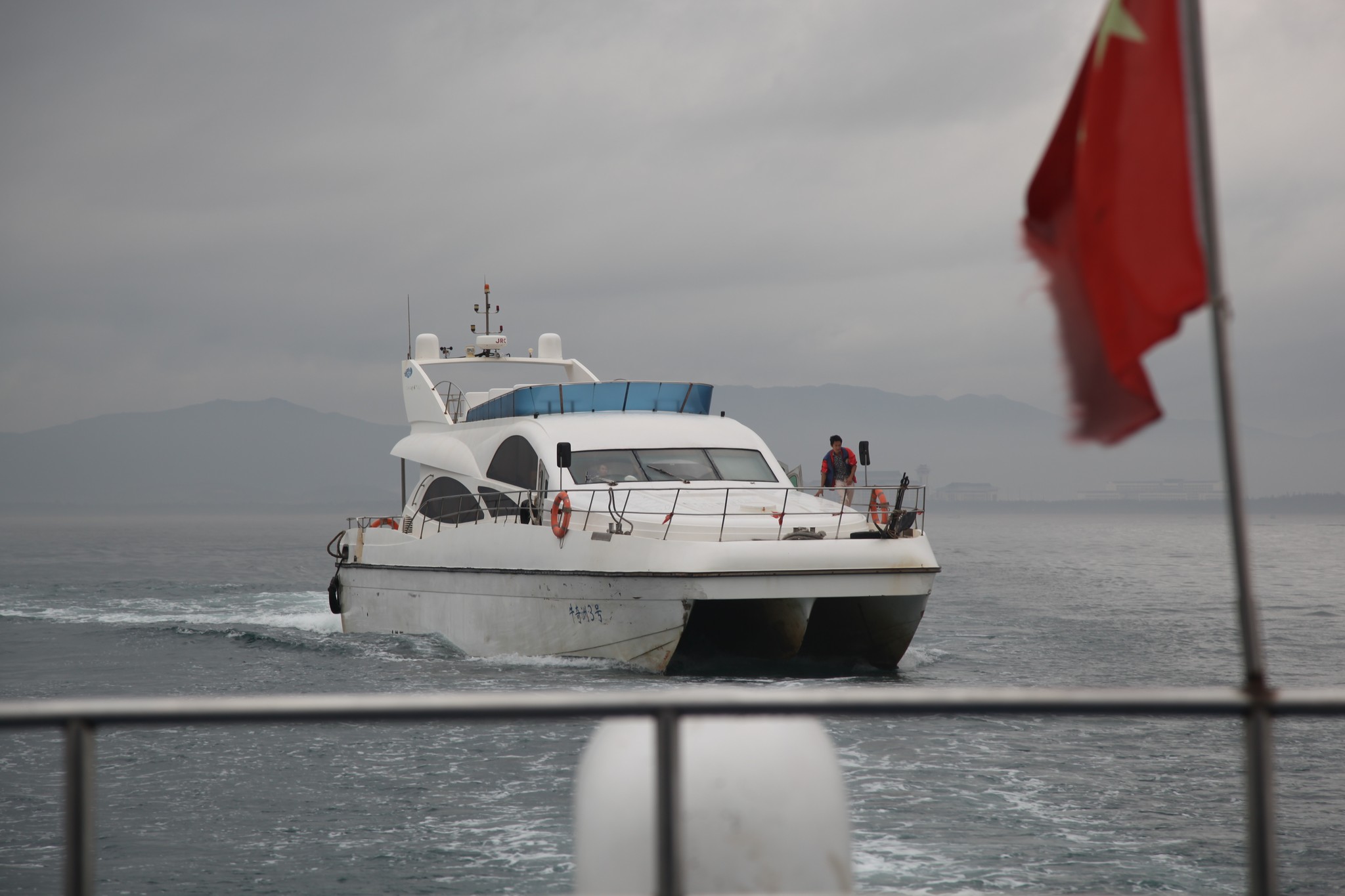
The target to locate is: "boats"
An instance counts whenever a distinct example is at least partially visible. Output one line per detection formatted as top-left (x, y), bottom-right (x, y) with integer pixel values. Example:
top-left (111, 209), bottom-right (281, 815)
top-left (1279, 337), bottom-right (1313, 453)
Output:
top-left (326, 282), bottom-right (941, 680)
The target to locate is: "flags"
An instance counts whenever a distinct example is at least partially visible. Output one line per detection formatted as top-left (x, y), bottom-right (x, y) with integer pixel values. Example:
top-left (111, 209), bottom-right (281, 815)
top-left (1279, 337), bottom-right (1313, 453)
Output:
top-left (1018, 1), bottom-right (1225, 448)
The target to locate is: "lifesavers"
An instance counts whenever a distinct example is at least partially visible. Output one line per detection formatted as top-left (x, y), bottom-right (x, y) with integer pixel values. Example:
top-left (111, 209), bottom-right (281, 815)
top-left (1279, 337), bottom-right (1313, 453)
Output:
top-left (870, 489), bottom-right (889, 524)
top-left (550, 491), bottom-right (572, 539)
top-left (369, 518), bottom-right (399, 531)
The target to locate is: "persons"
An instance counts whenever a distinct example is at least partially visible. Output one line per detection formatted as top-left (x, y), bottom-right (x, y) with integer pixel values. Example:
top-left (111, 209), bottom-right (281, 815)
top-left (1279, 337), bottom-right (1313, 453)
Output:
top-left (814, 435), bottom-right (857, 507)
top-left (588, 463), bottom-right (608, 484)
